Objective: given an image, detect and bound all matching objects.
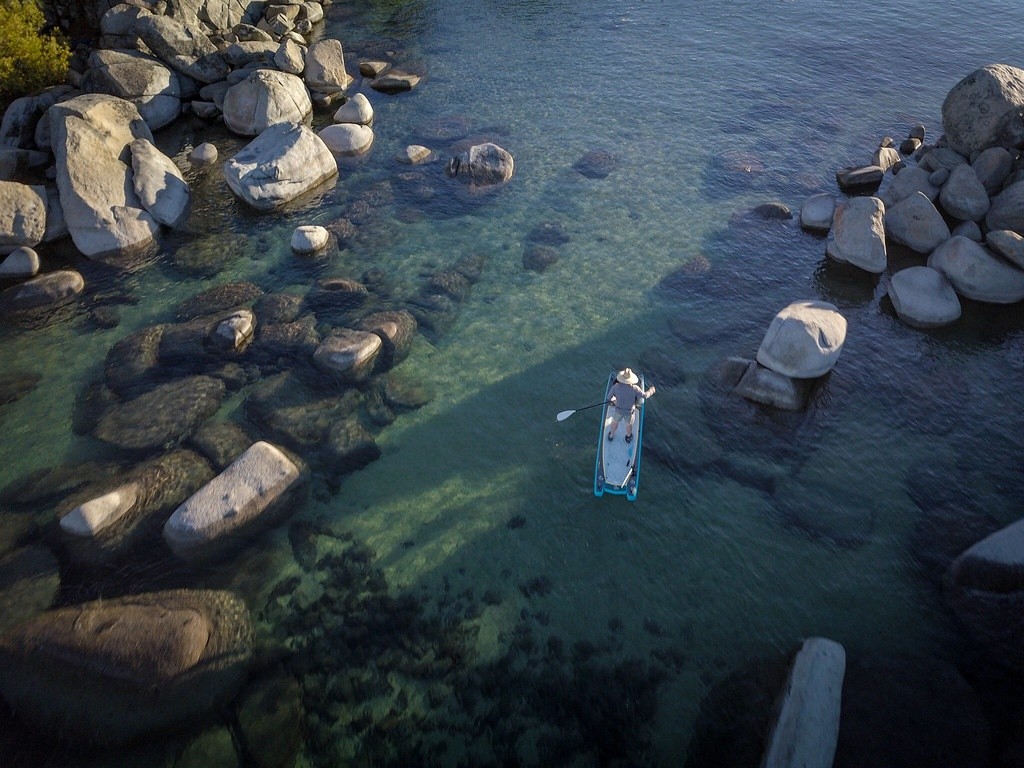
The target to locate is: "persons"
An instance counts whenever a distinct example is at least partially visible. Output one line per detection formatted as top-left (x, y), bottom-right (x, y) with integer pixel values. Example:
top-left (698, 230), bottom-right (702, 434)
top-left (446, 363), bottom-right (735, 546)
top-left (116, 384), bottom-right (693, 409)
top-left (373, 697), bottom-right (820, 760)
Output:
top-left (607, 370), bottom-right (656, 443)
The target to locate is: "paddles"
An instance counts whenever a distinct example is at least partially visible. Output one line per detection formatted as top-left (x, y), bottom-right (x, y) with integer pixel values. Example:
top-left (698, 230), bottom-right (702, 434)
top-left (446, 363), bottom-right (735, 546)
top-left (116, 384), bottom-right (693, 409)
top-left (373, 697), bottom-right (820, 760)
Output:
top-left (556, 400), bottom-right (617, 422)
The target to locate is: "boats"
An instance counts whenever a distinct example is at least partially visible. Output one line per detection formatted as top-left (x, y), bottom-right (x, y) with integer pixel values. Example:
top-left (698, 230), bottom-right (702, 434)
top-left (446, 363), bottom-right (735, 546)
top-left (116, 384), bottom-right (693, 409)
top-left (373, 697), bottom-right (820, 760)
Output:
top-left (556, 370), bottom-right (646, 503)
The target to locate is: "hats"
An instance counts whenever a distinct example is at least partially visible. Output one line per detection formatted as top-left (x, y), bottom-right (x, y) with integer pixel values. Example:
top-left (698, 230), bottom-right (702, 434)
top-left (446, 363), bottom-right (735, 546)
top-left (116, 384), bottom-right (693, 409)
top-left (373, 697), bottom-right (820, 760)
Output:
top-left (617, 368), bottom-right (638, 384)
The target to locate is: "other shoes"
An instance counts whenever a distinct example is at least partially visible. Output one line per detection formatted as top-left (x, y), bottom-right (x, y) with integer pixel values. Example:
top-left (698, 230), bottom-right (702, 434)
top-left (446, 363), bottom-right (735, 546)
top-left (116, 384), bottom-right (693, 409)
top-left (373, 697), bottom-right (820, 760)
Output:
top-left (608, 432), bottom-right (613, 442)
top-left (625, 434), bottom-right (633, 443)
